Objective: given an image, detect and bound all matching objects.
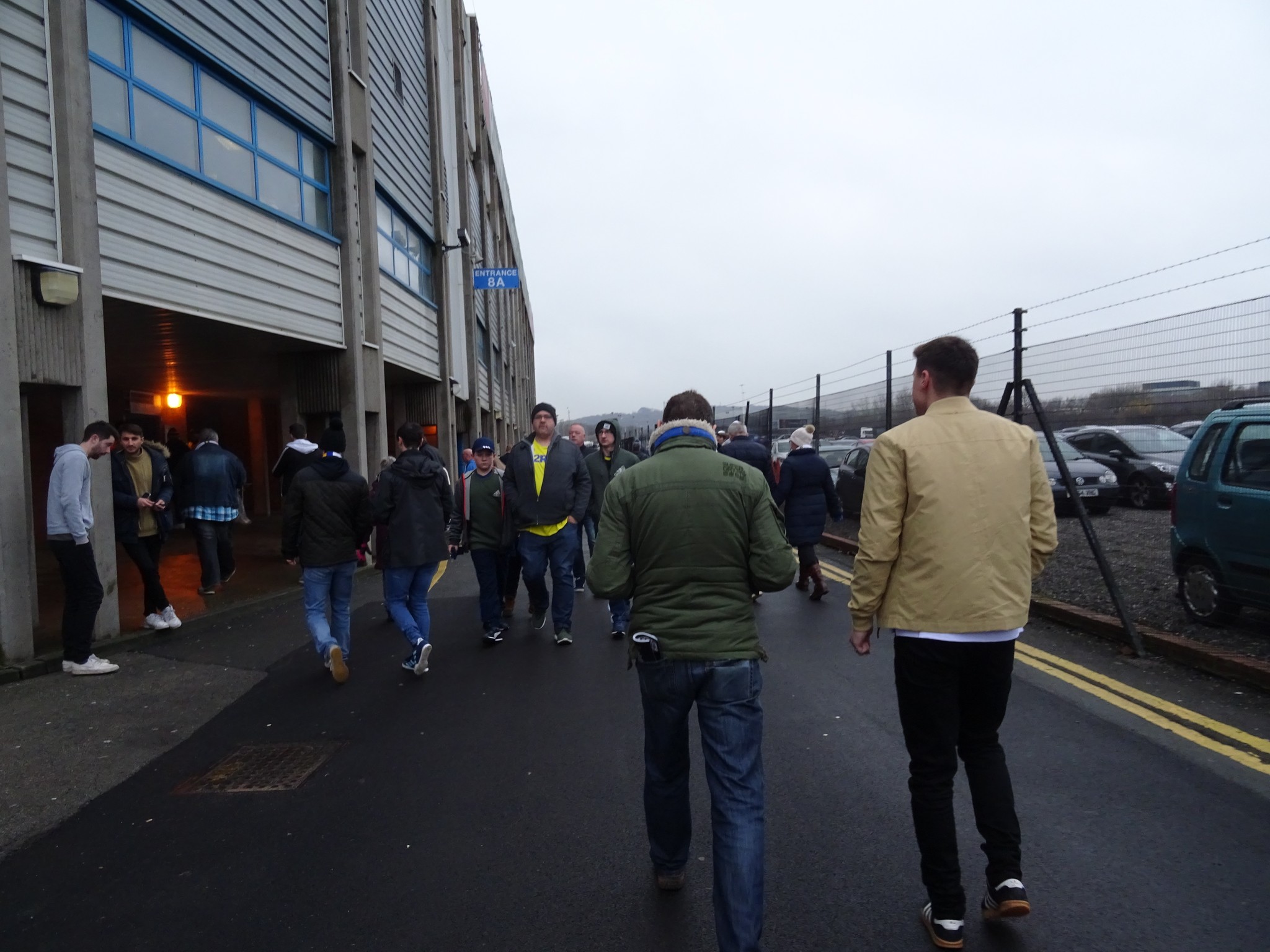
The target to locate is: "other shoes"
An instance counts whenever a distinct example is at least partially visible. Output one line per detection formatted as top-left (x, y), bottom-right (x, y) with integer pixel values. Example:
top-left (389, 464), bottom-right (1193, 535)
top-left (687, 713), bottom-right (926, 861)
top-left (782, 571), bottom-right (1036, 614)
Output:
top-left (503, 596), bottom-right (515, 617)
top-left (402, 640), bottom-right (433, 674)
top-left (573, 575), bottom-right (585, 592)
top-left (653, 864), bottom-right (687, 889)
top-left (197, 586), bottom-right (215, 594)
top-left (221, 565), bottom-right (236, 582)
top-left (325, 645), bottom-right (349, 683)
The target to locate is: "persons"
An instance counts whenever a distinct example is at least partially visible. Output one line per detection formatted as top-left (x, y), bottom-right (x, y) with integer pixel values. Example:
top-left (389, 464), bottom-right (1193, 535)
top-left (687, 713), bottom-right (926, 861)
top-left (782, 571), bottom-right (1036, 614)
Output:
top-left (776, 423), bottom-right (843, 601)
top-left (271, 422), bottom-right (320, 565)
top-left (448, 437), bottom-right (523, 641)
top-left (584, 418), bottom-right (641, 635)
top-left (355, 421), bottom-right (456, 675)
top-left (631, 437), bottom-right (650, 462)
top-left (280, 418), bottom-right (370, 683)
top-left (584, 391), bottom-right (798, 952)
top-left (848, 335), bottom-right (1060, 950)
top-left (174, 427), bottom-right (247, 594)
top-left (503, 401), bottom-right (593, 645)
top-left (713, 426), bottom-right (779, 469)
top-left (568, 422), bottom-right (601, 594)
top-left (718, 420), bottom-right (777, 512)
top-left (47, 420), bottom-right (119, 675)
top-left (111, 423), bottom-right (182, 630)
top-left (111, 423), bottom-right (251, 531)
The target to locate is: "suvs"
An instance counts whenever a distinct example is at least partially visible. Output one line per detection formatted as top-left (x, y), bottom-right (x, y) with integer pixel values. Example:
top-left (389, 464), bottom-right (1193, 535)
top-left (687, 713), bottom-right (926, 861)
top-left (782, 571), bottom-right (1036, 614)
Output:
top-left (1170, 396), bottom-right (1268, 627)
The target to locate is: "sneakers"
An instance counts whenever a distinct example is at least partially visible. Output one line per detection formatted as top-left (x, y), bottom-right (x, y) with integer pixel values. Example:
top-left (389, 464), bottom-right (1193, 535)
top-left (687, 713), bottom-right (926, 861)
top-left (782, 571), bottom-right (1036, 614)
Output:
top-left (62, 653), bottom-right (119, 675)
top-left (531, 613), bottom-right (546, 629)
top-left (920, 898), bottom-right (965, 948)
top-left (484, 624), bottom-right (509, 642)
top-left (980, 878), bottom-right (1031, 919)
top-left (554, 629), bottom-right (573, 644)
top-left (159, 604), bottom-right (182, 629)
top-left (611, 620), bottom-right (627, 635)
top-left (143, 613), bottom-right (169, 629)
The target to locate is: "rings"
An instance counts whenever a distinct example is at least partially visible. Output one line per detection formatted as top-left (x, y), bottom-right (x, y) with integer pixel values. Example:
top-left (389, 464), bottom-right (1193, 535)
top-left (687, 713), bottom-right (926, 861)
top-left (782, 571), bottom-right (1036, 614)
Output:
top-left (141, 505), bottom-right (145, 507)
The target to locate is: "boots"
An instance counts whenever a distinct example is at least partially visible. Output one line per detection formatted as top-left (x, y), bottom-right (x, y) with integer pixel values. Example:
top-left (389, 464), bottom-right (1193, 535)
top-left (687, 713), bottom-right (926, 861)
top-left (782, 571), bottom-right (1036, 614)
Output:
top-left (796, 564), bottom-right (828, 601)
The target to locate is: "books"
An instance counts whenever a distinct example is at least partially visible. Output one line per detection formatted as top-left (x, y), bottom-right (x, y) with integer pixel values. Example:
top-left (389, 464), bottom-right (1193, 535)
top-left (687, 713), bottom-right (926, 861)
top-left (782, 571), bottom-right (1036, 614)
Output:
top-left (632, 631), bottom-right (671, 699)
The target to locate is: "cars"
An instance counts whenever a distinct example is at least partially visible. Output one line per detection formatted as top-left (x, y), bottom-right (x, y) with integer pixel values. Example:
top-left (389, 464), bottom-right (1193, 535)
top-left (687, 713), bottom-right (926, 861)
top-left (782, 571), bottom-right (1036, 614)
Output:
top-left (769, 433), bottom-right (877, 521)
top-left (1034, 419), bottom-right (1208, 518)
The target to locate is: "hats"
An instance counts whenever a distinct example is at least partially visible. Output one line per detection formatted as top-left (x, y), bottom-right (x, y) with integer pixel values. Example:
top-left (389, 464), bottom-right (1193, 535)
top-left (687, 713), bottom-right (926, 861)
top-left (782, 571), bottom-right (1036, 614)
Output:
top-left (531, 403), bottom-right (557, 426)
top-left (318, 419), bottom-right (346, 453)
top-left (790, 425), bottom-right (815, 446)
top-left (596, 421), bottom-right (616, 436)
top-left (472, 437), bottom-right (495, 454)
